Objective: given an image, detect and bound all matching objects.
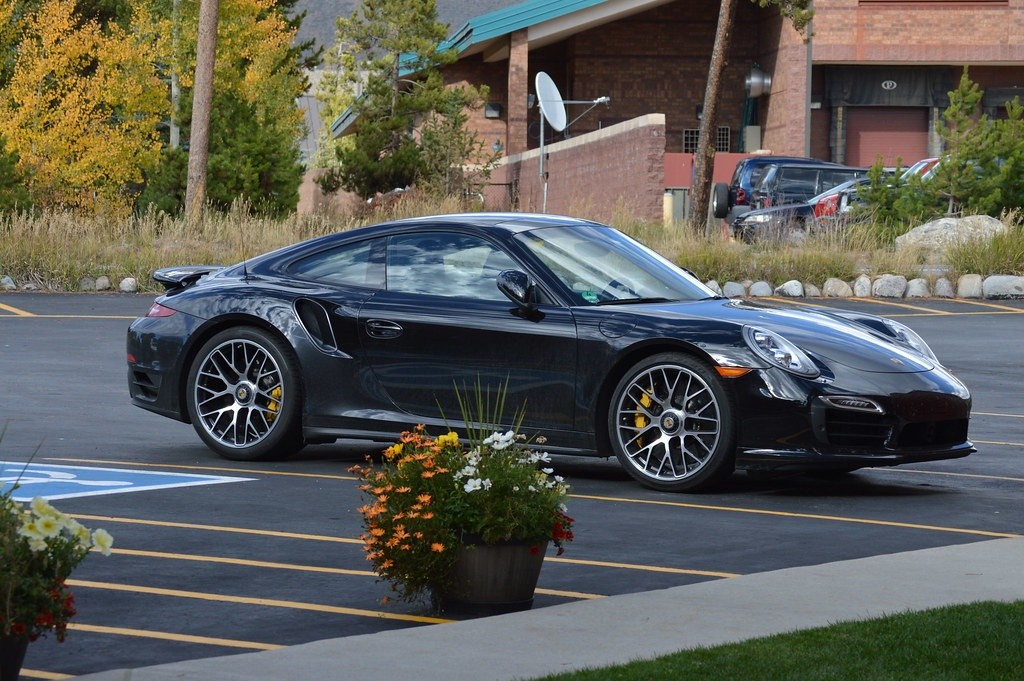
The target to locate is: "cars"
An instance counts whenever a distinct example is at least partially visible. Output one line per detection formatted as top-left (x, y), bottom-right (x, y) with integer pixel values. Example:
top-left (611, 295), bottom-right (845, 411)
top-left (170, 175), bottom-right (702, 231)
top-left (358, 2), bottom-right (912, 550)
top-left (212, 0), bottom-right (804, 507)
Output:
top-left (839, 153), bottom-right (1007, 226)
top-left (750, 162), bottom-right (891, 210)
top-left (733, 177), bottom-right (892, 245)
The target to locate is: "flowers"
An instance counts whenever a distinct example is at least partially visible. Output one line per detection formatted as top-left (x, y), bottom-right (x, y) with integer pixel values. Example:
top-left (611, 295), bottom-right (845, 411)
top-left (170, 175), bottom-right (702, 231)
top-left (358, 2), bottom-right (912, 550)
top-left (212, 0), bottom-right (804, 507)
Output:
top-left (0, 417), bottom-right (113, 644)
top-left (345, 370), bottom-right (575, 605)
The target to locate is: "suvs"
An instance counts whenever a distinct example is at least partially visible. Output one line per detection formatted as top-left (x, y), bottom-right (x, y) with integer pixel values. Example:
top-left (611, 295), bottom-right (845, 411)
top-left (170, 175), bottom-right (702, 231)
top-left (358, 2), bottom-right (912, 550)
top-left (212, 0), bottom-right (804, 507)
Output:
top-left (712, 154), bottom-right (849, 224)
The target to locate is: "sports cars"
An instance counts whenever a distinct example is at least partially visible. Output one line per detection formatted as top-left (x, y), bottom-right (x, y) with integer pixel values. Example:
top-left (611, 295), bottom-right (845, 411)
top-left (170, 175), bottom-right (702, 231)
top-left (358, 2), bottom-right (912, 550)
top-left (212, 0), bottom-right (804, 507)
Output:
top-left (125, 213), bottom-right (978, 494)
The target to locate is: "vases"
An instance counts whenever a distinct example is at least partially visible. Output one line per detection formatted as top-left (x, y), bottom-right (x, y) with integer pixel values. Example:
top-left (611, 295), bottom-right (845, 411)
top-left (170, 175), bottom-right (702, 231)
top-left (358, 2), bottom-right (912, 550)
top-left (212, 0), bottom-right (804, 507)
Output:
top-left (430, 529), bottom-right (549, 613)
top-left (0, 630), bottom-right (30, 681)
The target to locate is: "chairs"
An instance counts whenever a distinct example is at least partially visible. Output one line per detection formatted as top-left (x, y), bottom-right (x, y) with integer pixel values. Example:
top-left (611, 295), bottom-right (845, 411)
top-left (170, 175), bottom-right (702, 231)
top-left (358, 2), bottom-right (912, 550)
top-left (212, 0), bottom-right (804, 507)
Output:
top-left (403, 239), bottom-right (523, 302)
